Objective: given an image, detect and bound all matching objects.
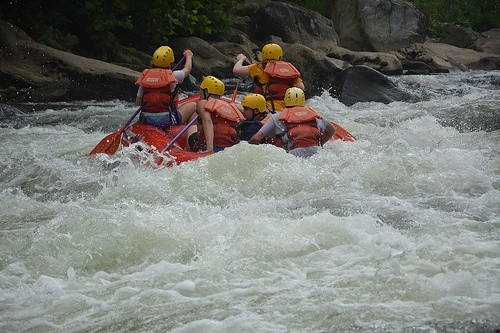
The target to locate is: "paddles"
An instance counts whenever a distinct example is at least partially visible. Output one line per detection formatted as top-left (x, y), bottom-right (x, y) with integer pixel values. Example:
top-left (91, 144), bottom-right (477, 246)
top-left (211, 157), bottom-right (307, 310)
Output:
top-left (244, 58), bottom-right (356, 142)
top-left (89, 48), bottom-right (187, 155)
top-left (231, 84), bottom-right (238, 102)
top-left (161, 115), bottom-right (198, 152)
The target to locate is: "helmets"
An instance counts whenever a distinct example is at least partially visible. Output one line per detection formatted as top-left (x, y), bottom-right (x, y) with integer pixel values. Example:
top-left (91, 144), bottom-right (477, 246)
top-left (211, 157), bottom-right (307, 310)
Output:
top-left (261, 44), bottom-right (283, 60)
top-left (284, 87), bottom-right (305, 107)
top-left (241, 93), bottom-right (267, 113)
top-left (152, 46), bottom-right (175, 68)
top-left (199, 75), bottom-right (225, 96)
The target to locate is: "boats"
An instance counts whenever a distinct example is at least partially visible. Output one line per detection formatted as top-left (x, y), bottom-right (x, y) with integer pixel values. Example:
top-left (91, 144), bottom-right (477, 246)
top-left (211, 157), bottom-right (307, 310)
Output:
top-left (119, 95), bottom-right (358, 172)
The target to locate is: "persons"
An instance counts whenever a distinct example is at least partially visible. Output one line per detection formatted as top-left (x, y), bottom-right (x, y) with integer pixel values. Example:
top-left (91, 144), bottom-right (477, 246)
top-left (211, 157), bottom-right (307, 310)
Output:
top-left (249, 87), bottom-right (335, 158)
top-left (234, 43), bottom-right (305, 100)
top-left (237, 93), bottom-right (284, 147)
top-left (188, 76), bottom-right (244, 153)
top-left (135, 46), bottom-right (197, 130)
